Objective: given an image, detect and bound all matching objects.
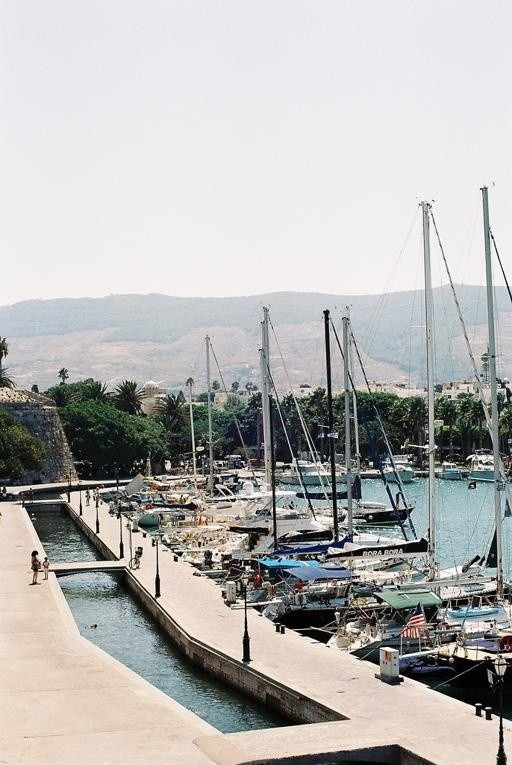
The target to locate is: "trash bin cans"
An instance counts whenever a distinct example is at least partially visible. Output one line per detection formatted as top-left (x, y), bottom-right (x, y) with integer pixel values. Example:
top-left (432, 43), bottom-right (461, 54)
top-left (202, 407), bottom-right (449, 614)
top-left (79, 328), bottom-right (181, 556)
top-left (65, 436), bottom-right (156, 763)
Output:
top-left (225, 581), bottom-right (239, 607)
top-left (380, 647), bottom-right (400, 685)
top-left (132, 519), bottom-right (139, 532)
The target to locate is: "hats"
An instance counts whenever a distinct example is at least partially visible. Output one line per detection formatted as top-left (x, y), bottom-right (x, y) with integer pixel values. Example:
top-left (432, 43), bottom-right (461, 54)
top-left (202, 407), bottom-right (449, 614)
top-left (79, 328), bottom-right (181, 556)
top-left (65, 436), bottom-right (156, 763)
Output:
top-left (44, 557), bottom-right (48, 559)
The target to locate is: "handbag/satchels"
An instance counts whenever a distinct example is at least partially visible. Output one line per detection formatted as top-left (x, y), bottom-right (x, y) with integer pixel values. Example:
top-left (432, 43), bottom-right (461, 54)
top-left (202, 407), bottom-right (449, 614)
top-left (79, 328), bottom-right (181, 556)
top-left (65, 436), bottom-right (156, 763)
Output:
top-left (34, 561), bottom-right (41, 569)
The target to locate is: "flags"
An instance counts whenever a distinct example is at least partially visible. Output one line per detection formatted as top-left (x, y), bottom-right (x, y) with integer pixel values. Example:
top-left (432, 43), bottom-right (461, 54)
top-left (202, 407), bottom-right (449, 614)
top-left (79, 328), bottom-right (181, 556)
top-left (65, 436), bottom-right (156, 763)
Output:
top-left (401, 601), bottom-right (425, 638)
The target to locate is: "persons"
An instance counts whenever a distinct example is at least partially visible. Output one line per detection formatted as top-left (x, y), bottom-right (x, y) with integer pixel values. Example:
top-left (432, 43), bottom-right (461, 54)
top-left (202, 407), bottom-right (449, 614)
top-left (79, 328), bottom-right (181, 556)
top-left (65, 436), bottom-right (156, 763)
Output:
top-left (43, 557), bottom-right (50, 579)
top-left (20, 488), bottom-right (33, 502)
top-left (109, 502), bottom-right (121, 519)
top-left (253, 569), bottom-right (262, 588)
top-left (294, 579), bottom-right (307, 605)
top-left (204, 550), bottom-right (214, 569)
top-left (86, 486), bottom-right (100, 504)
top-left (31, 551), bottom-right (39, 583)
top-left (173, 510), bottom-right (179, 526)
top-left (180, 495), bottom-right (184, 503)
top-left (2, 486), bottom-right (7, 501)
